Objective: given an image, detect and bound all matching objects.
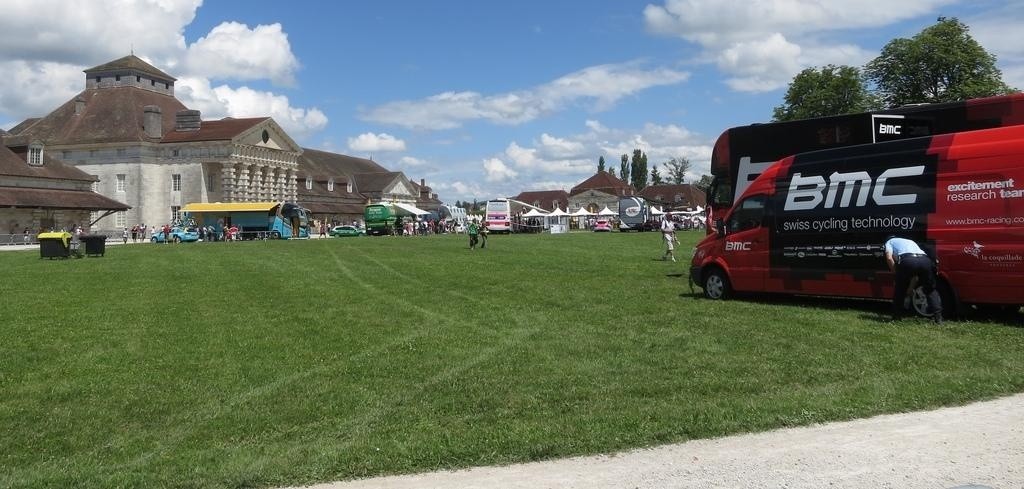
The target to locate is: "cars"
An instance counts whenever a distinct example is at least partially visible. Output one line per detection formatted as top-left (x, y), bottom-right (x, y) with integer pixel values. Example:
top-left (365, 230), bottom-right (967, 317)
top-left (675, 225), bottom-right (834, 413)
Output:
top-left (329, 225), bottom-right (369, 238)
top-left (150, 227), bottom-right (200, 244)
top-left (452, 224), bottom-right (467, 233)
top-left (594, 220), bottom-right (613, 232)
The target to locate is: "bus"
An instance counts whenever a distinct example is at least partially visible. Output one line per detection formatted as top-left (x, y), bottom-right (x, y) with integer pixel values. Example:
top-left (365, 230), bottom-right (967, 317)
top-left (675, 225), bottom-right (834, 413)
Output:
top-left (485, 198), bottom-right (551, 234)
top-left (618, 196), bottom-right (705, 232)
top-left (690, 123), bottom-right (1024, 318)
top-left (365, 202), bottom-right (417, 234)
top-left (700, 91), bottom-right (1023, 237)
top-left (179, 202), bottom-right (312, 239)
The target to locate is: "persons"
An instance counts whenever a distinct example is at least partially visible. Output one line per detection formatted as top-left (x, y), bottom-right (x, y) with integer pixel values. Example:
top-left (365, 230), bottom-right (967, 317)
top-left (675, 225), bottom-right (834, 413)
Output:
top-left (352, 219), bottom-right (357, 227)
top-left (883, 234), bottom-right (945, 325)
top-left (468, 218), bottom-right (479, 250)
top-left (659, 213), bottom-right (680, 263)
top-left (401, 217), bottom-right (446, 236)
top-left (121, 227), bottom-right (128, 243)
top-left (150, 224), bottom-right (155, 238)
top-left (160, 224), bottom-right (165, 232)
top-left (535, 219), bottom-right (540, 225)
top-left (527, 219), bottom-right (531, 225)
top-left (317, 222), bottom-right (326, 240)
top-left (170, 224), bottom-right (179, 246)
top-left (477, 222), bottom-right (489, 249)
top-left (195, 223), bottom-right (242, 241)
top-left (60, 226), bottom-right (66, 232)
top-left (75, 225), bottom-right (82, 235)
top-left (163, 224), bottom-right (169, 244)
top-left (131, 222), bottom-right (147, 243)
top-left (22, 227), bottom-right (31, 245)
top-left (327, 222), bottom-right (331, 232)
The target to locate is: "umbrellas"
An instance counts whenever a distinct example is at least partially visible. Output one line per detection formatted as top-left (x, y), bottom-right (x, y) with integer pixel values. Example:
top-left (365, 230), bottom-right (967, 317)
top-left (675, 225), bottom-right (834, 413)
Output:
top-left (546, 207), bottom-right (570, 224)
top-left (570, 206), bottom-right (596, 225)
top-left (647, 206), bottom-right (664, 221)
top-left (597, 206), bottom-right (619, 220)
top-left (521, 208), bottom-right (546, 225)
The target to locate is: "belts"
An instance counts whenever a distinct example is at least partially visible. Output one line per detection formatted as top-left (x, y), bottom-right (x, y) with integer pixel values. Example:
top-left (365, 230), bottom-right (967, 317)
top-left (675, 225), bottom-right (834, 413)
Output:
top-left (901, 254), bottom-right (923, 258)
